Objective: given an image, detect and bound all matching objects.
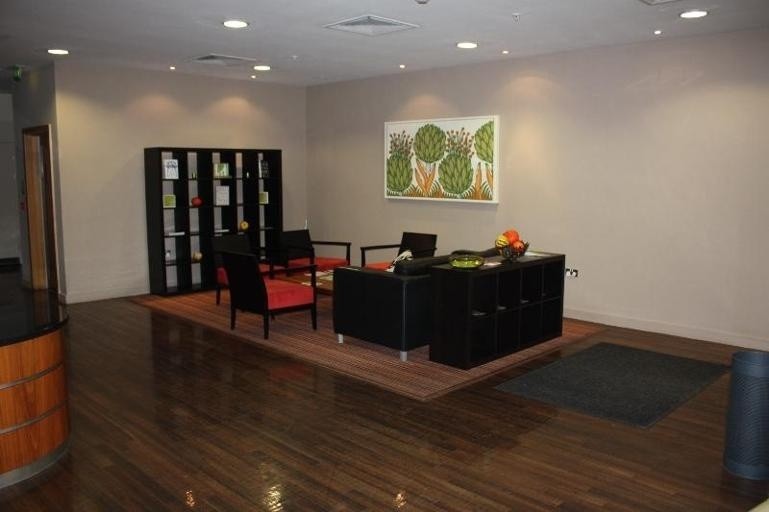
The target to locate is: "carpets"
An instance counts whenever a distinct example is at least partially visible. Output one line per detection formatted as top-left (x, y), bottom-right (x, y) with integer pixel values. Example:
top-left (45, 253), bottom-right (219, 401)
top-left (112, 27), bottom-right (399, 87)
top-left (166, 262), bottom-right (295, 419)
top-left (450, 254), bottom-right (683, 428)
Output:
top-left (492, 342), bottom-right (732, 430)
top-left (124, 287), bottom-right (611, 405)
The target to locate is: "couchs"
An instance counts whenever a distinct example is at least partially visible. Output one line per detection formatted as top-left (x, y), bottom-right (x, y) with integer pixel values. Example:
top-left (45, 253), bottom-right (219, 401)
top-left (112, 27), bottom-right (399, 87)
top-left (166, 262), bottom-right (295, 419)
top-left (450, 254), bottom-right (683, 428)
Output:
top-left (333, 247), bottom-right (501, 362)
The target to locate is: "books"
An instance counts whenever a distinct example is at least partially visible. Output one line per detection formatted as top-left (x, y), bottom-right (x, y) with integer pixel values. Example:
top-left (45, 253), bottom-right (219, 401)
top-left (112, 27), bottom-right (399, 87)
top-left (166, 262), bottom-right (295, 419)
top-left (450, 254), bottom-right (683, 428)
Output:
top-left (166, 231), bottom-right (186, 237)
top-left (214, 229), bottom-right (231, 234)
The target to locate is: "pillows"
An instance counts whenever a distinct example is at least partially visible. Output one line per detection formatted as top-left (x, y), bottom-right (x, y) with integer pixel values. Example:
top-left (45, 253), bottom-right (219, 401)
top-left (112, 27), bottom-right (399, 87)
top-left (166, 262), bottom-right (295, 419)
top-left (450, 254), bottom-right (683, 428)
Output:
top-left (385, 249), bottom-right (414, 273)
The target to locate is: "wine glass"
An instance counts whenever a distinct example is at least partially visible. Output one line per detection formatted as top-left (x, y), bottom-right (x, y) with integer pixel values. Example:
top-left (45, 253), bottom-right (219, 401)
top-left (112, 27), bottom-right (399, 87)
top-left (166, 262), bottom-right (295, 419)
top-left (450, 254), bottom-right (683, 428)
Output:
top-left (497, 242), bottom-right (529, 263)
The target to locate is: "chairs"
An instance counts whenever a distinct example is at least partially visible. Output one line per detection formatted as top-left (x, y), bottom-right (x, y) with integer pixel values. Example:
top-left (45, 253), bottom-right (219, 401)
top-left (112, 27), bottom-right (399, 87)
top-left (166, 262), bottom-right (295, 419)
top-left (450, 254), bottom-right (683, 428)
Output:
top-left (208, 229), bottom-right (352, 340)
top-left (359, 232), bottom-right (437, 271)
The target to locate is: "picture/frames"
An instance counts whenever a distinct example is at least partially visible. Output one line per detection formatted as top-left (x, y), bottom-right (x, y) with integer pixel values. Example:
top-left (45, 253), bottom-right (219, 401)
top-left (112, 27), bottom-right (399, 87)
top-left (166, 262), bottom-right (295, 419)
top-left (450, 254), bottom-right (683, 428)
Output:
top-left (384, 114), bottom-right (499, 204)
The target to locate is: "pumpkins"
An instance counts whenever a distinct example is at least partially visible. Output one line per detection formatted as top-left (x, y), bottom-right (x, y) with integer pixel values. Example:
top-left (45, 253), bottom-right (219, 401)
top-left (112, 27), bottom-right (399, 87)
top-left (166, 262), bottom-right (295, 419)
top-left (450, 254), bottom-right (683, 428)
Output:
top-left (495, 230), bottom-right (524, 250)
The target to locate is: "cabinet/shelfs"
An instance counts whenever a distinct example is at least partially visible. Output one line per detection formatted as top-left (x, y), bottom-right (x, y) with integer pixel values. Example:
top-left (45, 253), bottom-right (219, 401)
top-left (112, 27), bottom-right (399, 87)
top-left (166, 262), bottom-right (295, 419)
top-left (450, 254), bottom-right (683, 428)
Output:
top-left (145, 147), bottom-right (284, 298)
top-left (430, 250), bottom-right (566, 371)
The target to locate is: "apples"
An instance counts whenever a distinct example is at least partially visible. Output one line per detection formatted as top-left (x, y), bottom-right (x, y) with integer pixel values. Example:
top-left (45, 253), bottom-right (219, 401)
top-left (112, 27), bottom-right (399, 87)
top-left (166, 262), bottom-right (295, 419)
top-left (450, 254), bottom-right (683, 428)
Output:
top-left (192, 198), bottom-right (202, 207)
top-left (241, 222), bottom-right (249, 230)
